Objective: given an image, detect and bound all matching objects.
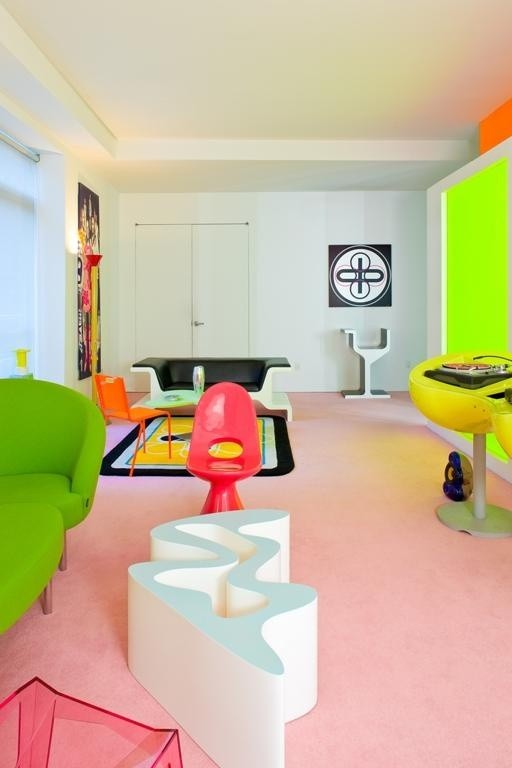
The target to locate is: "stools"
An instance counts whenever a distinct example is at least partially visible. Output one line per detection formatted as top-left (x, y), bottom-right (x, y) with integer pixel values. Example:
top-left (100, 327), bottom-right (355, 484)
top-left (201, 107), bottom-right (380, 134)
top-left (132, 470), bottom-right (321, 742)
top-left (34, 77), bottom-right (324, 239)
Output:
top-left (0, 675), bottom-right (184, 768)
top-left (341, 328), bottom-right (393, 399)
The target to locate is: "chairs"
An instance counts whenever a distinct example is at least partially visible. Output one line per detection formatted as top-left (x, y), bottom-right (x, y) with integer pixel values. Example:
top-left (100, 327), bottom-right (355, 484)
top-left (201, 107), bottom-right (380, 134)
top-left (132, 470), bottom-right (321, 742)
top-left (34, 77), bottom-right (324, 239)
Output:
top-left (0, 377), bottom-right (106, 644)
top-left (186, 382), bottom-right (264, 534)
top-left (93, 372), bottom-right (173, 478)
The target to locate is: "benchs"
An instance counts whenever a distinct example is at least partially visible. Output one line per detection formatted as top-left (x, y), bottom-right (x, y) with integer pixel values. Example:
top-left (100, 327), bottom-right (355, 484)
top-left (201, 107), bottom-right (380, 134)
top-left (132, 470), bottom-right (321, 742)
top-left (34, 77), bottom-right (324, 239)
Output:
top-left (130, 355), bottom-right (291, 403)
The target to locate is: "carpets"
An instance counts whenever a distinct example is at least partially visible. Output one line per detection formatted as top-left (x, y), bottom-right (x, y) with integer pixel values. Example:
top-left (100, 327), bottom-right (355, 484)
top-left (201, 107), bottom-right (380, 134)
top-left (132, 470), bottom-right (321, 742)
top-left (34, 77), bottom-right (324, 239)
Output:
top-left (103, 413), bottom-right (298, 479)
